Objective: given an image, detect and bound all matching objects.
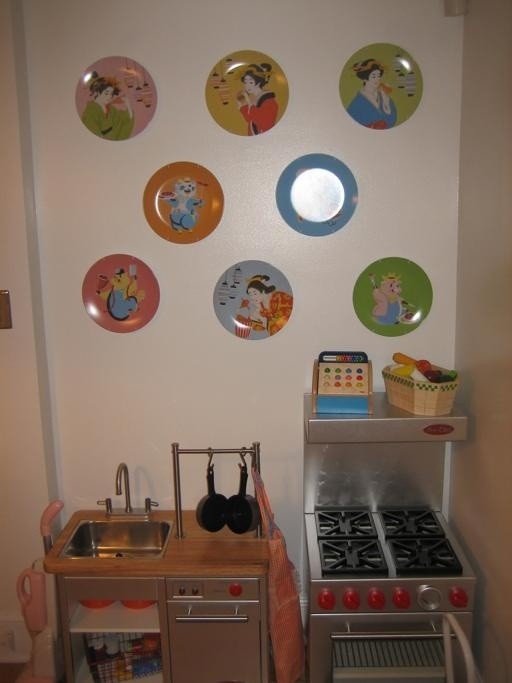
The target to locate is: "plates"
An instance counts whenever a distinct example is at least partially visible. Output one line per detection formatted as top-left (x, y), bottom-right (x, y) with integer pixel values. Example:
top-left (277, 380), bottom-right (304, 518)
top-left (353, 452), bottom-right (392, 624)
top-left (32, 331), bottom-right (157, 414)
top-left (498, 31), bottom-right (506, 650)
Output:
top-left (140, 159), bottom-right (225, 243)
top-left (201, 48), bottom-right (290, 135)
top-left (75, 55), bottom-right (159, 142)
top-left (352, 256), bottom-right (433, 338)
top-left (210, 258), bottom-right (294, 342)
top-left (81, 252), bottom-right (161, 333)
top-left (271, 152), bottom-right (360, 239)
top-left (337, 43), bottom-right (427, 130)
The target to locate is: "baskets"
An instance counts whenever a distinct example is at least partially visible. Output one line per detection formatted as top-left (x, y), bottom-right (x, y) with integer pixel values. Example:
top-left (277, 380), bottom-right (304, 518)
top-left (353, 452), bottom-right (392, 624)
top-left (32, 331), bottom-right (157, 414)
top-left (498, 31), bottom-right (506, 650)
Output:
top-left (381, 359), bottom-right (462, 418)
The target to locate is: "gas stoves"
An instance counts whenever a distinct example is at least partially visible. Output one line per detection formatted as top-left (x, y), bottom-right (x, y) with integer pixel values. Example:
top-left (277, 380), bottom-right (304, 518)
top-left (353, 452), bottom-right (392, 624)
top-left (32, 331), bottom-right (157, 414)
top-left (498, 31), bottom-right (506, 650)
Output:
top-left (314, 507), bottom-right (466, 577)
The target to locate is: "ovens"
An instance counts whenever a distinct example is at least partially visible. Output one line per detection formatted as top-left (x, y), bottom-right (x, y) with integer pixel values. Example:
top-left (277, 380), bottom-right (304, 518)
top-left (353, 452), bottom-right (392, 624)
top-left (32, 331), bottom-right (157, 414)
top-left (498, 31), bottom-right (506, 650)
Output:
top-left (308, 612), bottom-right (477, 681)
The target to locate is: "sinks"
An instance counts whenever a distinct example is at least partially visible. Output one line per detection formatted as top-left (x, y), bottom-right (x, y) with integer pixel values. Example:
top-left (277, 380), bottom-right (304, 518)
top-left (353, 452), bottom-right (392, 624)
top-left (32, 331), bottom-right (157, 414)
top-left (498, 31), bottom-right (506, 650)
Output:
top-left (61, 518), bottom-right (175, 561)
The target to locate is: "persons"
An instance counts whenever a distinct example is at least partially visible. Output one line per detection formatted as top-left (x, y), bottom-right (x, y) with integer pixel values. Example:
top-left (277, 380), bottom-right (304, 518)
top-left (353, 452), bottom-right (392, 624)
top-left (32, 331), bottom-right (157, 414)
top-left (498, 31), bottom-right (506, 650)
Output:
top-left (236, 63), bottom-right (278, 136)
top-left (82, 71), bottom-right (134, 140)
top-left (247, 275), bottom-right (293, 336)
top-left (347, 59), bottom-right (396, 130)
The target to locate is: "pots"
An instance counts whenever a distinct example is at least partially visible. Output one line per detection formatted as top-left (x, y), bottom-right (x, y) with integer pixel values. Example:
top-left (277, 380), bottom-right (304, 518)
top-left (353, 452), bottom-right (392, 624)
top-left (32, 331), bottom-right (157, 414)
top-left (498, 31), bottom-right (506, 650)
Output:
top-left (195, 467), bottom-right (228, 533)
top-left (224, 468), bottom-right (262, 532)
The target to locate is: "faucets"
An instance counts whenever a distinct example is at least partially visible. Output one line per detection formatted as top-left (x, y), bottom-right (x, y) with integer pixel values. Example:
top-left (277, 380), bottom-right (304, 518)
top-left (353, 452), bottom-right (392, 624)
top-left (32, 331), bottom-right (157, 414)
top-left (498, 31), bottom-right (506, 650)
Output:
top-left (115, 461), bottom-right (133, 513)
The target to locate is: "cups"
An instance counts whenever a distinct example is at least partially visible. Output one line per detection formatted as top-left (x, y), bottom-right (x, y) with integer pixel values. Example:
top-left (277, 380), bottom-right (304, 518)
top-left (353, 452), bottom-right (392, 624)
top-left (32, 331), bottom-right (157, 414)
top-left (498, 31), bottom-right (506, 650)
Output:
top-left (143, 637), bottom-right (161, 659)
top-left (104, 636), bottom-right (120, 658)
